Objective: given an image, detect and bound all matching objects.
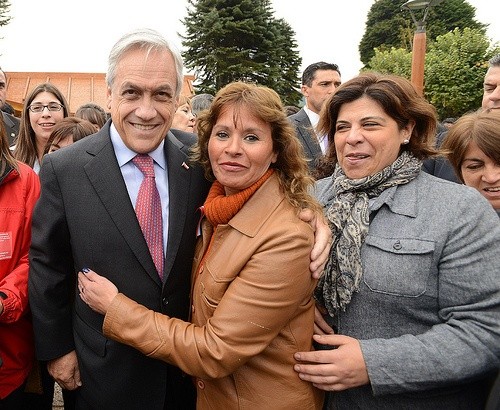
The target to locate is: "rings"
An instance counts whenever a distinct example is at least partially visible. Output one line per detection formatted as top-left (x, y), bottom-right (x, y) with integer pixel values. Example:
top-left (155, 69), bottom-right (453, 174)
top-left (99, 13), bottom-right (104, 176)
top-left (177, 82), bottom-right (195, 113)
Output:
top-left (82, 287), bottom-right (84, 293)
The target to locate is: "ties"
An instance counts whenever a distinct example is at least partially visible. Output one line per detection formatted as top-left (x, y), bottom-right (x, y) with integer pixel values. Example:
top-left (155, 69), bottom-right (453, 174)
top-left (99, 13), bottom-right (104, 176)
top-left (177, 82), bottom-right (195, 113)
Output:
top-left (130, 154), bottom-right (165, 280)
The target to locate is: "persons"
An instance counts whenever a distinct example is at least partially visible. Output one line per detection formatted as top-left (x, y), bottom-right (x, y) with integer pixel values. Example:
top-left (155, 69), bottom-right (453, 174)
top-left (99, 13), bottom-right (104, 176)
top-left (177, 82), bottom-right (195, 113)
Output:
top-left (294, 77), bottom-right (500, 410)
top-left (0, 110), bottom-right (41, 410)
top-left (282, 62), bottom-right (342, 174)
top-left (78, 81), bottom-right (325, 410)
top-left (29, 29), bottom-right (332, 410)
top-left (170, 93), bottom-right (216, 134)
top-left (416, 53), bottom-right (500, 218)
top-left (0, 68), bottom-right (107, 174)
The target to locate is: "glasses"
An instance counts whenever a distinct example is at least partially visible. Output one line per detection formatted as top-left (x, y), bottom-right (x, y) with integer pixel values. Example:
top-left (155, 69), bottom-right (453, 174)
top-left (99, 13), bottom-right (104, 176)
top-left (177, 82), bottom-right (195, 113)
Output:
top-left (28, 103), bottom-right (65, 113)
